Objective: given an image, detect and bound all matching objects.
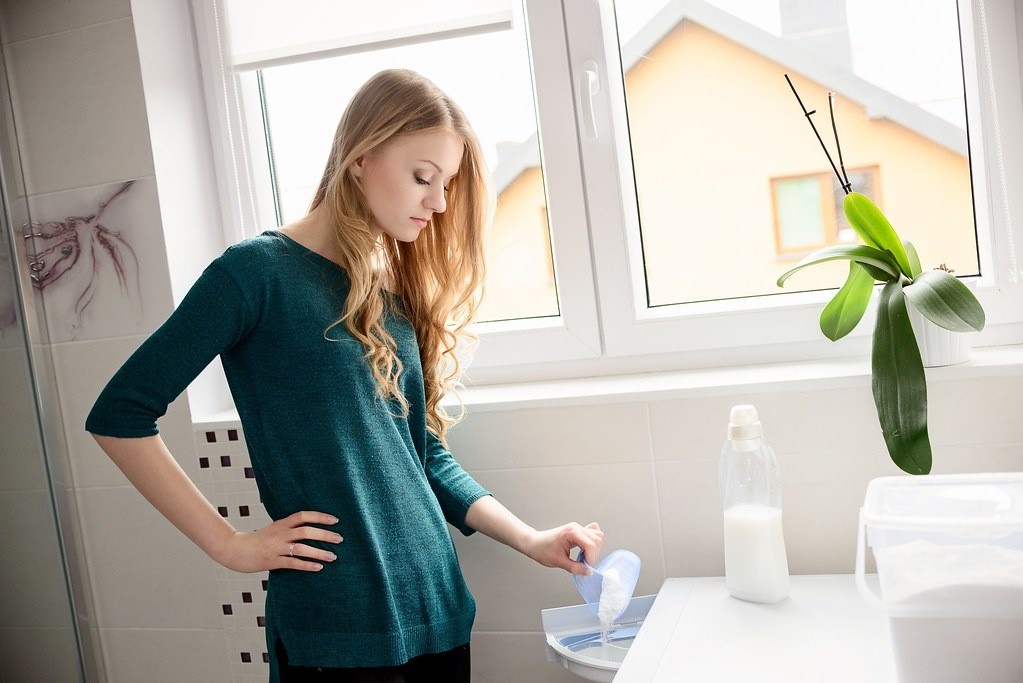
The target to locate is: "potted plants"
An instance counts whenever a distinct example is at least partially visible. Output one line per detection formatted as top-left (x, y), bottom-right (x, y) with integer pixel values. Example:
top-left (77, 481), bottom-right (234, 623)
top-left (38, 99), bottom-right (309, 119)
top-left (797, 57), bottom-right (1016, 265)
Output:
top-left (769, 69), bottom-right (986, 476)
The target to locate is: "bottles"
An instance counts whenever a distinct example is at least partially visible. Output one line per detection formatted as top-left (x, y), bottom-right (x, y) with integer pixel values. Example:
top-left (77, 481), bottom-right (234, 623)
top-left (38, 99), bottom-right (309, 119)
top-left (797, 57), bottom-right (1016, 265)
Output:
top-left (718, 405), bottom-right (790, 603)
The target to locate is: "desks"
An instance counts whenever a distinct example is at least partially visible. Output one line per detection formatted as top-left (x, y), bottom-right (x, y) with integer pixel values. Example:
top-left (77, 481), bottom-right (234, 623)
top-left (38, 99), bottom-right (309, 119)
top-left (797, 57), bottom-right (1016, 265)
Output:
top-left (607, 570), bottom-right (906, 683)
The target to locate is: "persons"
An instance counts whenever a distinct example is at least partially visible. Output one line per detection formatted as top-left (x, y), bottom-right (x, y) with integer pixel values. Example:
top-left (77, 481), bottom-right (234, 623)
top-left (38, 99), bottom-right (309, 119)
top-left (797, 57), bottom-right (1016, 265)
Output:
top-left (82, 66), bottom-right (604, 683)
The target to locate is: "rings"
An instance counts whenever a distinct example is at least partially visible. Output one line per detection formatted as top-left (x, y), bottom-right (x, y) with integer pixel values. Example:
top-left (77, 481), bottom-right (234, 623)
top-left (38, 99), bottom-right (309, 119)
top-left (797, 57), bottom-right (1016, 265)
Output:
top-left (289, 543), bottom-right (295, 556)
